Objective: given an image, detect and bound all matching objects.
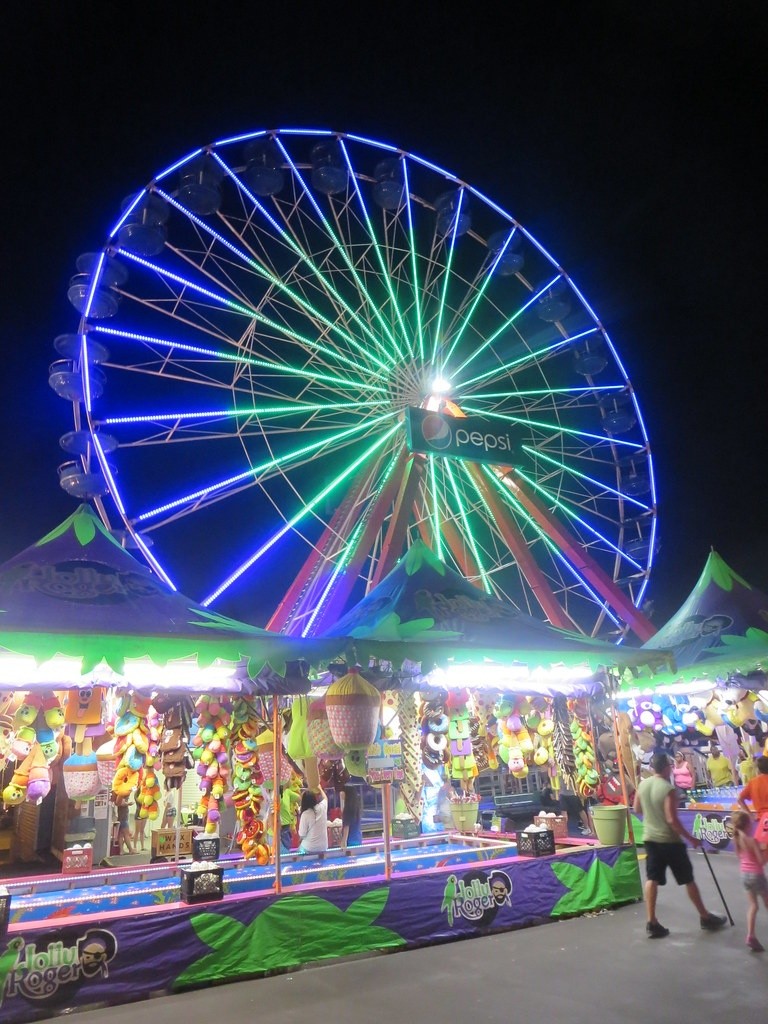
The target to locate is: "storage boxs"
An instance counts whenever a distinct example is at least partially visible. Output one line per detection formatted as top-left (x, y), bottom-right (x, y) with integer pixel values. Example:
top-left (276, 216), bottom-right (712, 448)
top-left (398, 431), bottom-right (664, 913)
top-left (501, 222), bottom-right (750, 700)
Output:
top-left (193, 834), bottom-right (220, 860)
top-left (152, 827), bottom-right (193, 857)
top-left (61, 843), bottom-right (93, 874)
top-left (515, 823), bottom-right (555, 857)
top-left (393, 813), bottom-right (421, 837)
top-left (533, 810), bottom-right (568, 837)
top-left (327, 820), bottom-right (343, 847)
top-left (179, 866), bottom-right (223, 905)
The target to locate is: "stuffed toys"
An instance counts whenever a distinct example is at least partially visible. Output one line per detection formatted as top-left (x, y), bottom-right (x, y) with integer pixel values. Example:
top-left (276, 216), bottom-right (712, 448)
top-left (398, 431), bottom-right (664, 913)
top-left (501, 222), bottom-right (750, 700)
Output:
top-left (0, 690), bottom-right (66, 813)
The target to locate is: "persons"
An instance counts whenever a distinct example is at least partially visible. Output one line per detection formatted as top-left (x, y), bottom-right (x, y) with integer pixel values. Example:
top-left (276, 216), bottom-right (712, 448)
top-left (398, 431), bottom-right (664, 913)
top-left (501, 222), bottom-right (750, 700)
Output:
top-left (438, 778), bottom-right (455, 812)
top-left (634, 747), bottom-right (736, 934)
top-left (738, 739), bottom-right (768, 868)
top-left (732, 811), bottom-right (768, 950)
top-left (117, 789), bottom-right (140, 855)
top-left (541, 774), bottom-right (597, 836)
top-left (643, 615), bottom-right (733, 650)
top-left (298, 784), bottom-right (328, 854)
top-left (340, 786), bottom-right (362, 851)
top-left (279, 785), bottom-right (302, 850)
top-left (160, 789), bottom-right (176, 829)
top-left (134, 788), bottom-right (148, 851)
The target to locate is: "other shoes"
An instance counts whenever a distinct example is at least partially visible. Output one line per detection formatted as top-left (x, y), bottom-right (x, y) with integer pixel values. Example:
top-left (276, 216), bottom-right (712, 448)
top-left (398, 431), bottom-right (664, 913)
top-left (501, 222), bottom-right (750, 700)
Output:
top-left (581, 829), bottom-right (596, 836)
top-left (577, 821), bottom-right (585, 829)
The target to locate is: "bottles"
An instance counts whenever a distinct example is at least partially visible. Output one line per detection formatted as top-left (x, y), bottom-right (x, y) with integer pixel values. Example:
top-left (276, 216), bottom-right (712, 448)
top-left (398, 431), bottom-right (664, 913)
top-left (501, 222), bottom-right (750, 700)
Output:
top-left (687, 786), bottom-right (742, 803)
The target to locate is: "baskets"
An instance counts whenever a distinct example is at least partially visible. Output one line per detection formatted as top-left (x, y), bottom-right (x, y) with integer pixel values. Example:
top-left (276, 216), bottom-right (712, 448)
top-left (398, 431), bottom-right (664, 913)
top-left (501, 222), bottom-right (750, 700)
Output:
top-left (62, 848), bottom-right (92, 874)
top-left (516, 830), bottom-right (556, 857)
top-left (192, 837), bottom-right (219, 861)
top-left (534, 815), bottom-right (568, 838)
top-left (180, 865), bottom-right (224, 904)
top-left (0, 895), bottom-right (12, 935)
top-left (392, 817), bottom-right (418, 838)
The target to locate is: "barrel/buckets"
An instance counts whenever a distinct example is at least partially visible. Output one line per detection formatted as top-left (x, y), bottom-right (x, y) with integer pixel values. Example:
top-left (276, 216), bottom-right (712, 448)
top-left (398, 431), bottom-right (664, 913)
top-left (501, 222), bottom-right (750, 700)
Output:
top-left (589, 806), bottom-right (627, 845)
top-left (451, 803), bottom-right (478, 831)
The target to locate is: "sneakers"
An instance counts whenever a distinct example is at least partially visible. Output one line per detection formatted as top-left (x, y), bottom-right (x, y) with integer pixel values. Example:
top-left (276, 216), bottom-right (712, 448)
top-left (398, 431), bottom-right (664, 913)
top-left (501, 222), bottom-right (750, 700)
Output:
top-left (646, 919), bottom-right (669, 938)
top-left (700, 913), bottom-right (727, 929)
top-left (746, 935), bottom-right (764, 950)
